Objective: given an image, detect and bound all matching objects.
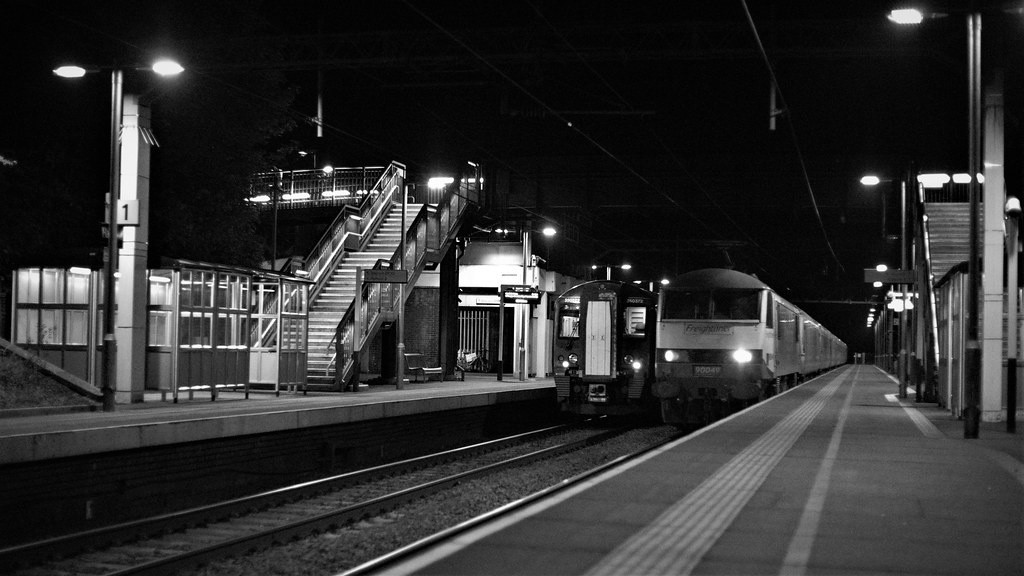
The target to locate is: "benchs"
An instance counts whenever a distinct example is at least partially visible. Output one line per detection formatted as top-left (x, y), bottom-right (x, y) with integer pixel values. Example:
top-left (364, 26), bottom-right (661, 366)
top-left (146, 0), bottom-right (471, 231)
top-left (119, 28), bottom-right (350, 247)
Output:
top-left (403, 353), bottom-right (443, 383)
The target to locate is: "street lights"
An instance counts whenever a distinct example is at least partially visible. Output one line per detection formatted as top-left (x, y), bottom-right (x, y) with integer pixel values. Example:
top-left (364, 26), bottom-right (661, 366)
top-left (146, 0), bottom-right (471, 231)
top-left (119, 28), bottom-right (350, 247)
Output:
top-left (860, 176), bottom-right (909, 399)
top-left (886, 6), bottom-right (979, 442)
top-left (271, 167), bottom-right (334, 272)
top-left (866, 264), bottom-right (900, 378)
top-left (519, 228), bottom-right (557, 381)
top-left (53, 59), bottom-right (187, 409)
top-left (592, 264), bottom-right (632, 280)
top-left (396, 175), bottom-right (459, 391)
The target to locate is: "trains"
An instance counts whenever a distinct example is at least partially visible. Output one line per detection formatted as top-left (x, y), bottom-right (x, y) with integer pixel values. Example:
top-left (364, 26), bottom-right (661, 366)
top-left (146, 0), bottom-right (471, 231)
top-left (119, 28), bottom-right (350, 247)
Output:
top-left (654, 268), bottom-right (848, 433)
top-left (552, 278), bottom-right (659, 419)
